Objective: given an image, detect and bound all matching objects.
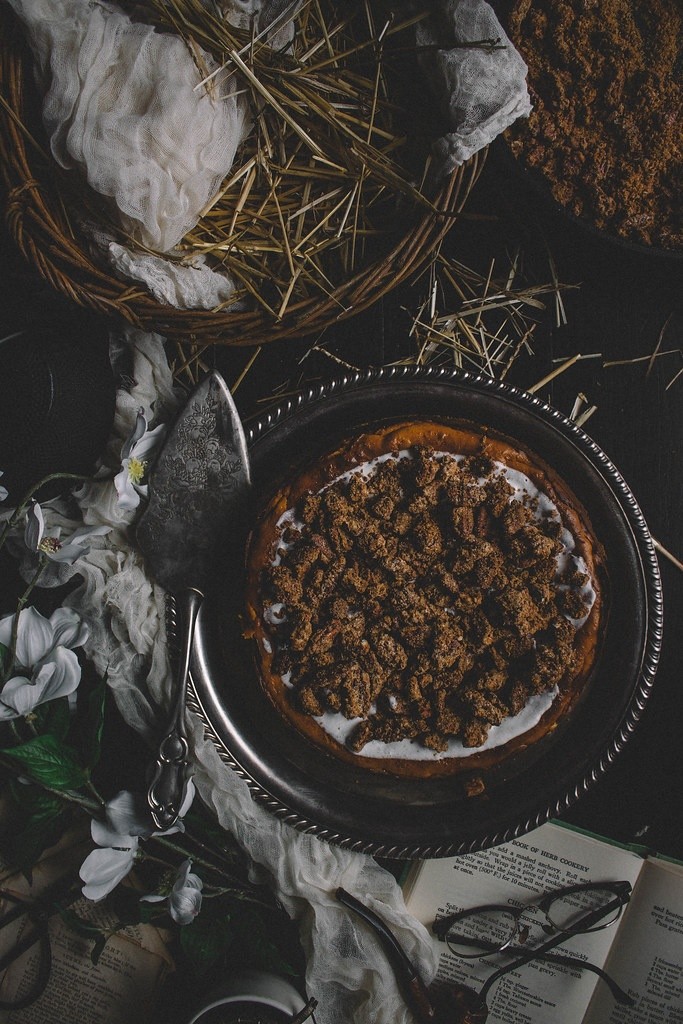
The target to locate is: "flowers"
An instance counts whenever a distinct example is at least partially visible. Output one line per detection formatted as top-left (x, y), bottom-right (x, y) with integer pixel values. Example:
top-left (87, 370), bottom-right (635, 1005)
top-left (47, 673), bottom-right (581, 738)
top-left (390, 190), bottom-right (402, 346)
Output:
top-left (0, 404), bottom-right (315, 999)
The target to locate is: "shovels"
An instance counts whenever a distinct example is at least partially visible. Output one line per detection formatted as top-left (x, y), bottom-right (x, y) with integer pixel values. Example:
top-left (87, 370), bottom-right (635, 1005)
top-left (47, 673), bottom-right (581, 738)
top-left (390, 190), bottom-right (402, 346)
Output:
top-left (137, 369), bottom-right (254, 833)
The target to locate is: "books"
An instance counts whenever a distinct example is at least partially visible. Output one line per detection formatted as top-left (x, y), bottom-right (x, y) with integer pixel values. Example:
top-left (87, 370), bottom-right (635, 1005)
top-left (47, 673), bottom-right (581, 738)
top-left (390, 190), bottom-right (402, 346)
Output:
top-left (392, 819), bottom-right (683, 1023)
top-left (0, 825), bottom-right (191, 1024)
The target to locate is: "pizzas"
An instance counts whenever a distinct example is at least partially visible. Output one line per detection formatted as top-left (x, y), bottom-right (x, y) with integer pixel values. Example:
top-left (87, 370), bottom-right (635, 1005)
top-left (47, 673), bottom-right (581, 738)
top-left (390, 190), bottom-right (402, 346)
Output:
top-left (242, 419), bottom-right (601, 780)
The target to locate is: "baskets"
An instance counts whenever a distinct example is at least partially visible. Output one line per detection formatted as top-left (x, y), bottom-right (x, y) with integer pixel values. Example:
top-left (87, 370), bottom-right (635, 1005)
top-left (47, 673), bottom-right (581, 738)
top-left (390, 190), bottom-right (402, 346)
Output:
top-left (4, 3), bottom-right (489, 341)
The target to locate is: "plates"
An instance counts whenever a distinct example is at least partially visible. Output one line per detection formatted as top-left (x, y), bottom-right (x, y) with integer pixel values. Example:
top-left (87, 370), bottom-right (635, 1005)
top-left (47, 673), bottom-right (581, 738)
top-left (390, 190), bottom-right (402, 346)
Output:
top-left (165, 363), bottom-right (663, 860)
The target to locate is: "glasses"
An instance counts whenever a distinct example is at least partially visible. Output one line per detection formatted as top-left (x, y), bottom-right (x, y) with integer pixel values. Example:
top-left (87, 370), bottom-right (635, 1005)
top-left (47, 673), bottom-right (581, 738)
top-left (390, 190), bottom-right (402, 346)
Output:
top-left (433, 879), bottom-right (634, 1008)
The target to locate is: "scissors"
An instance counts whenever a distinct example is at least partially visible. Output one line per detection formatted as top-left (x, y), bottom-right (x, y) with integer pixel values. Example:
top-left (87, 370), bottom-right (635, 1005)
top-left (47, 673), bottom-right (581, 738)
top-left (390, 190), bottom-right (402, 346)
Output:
top-left (0, 878), bottom-right (86, 1012)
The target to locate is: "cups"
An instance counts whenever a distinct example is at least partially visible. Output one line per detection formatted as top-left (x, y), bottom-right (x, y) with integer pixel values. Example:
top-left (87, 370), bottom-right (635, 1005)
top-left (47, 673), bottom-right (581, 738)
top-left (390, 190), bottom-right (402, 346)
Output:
top-left (186, 967), bottom-right (315, 1024)
